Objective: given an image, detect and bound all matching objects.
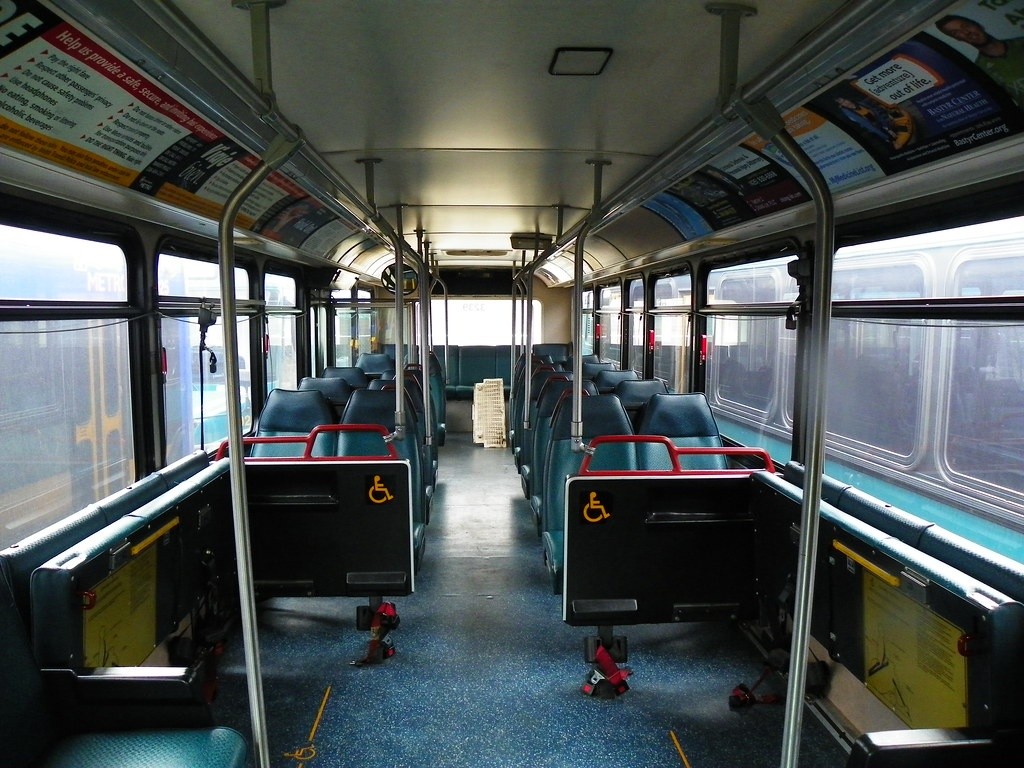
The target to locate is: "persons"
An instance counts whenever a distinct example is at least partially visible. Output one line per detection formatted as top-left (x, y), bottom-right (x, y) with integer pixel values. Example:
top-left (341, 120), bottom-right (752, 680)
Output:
top-left (935, 14), bottom-right (1024, 112)
top-left (707, 169), bottom-right (744, 196)
top-left (835, 96), bottom-right (899, 144)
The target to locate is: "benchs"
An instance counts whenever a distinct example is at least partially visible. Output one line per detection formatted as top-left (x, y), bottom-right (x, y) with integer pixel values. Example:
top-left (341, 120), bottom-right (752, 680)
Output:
top-left (0, 340), bottom-right (729, 768)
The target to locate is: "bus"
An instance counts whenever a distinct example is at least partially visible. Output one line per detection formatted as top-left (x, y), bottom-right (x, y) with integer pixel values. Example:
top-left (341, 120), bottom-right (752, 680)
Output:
top-left (582, 213), bottom-right (1024, 564)
top-left (0, 222), bottom-right (300, 549)
